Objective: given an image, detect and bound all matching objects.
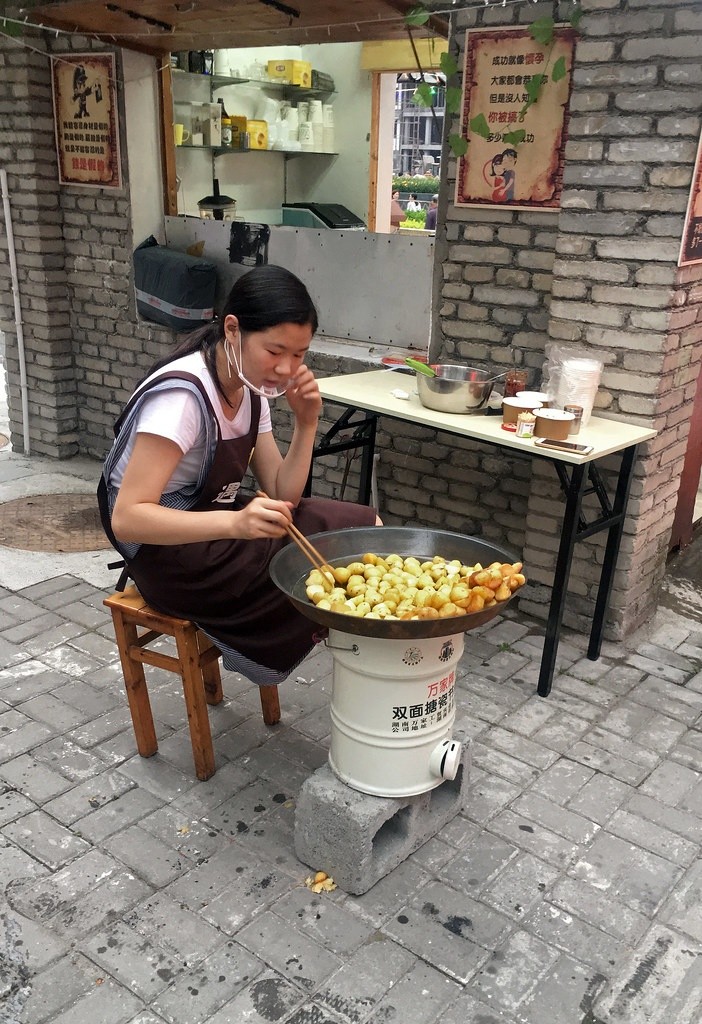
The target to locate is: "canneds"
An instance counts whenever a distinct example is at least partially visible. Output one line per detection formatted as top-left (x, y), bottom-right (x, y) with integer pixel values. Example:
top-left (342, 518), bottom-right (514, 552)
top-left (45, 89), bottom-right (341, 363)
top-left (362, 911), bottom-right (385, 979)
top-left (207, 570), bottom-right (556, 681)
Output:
top-left (504, 370), bottom-right (526, 398)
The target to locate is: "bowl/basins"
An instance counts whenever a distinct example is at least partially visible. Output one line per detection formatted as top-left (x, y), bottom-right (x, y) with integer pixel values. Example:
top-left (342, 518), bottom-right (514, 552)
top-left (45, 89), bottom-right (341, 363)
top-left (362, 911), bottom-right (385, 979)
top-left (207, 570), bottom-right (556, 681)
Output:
top-left (502, 361), bottom-right (600, 440)
top-left (416, 364), bottom-right (500, 414)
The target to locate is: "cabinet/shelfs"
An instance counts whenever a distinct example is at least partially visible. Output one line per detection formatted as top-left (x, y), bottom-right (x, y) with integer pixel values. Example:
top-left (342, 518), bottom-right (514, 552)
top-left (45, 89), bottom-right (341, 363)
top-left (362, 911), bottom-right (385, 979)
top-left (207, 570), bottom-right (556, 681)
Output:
top-left (170, 69), bottom-right (340, 160)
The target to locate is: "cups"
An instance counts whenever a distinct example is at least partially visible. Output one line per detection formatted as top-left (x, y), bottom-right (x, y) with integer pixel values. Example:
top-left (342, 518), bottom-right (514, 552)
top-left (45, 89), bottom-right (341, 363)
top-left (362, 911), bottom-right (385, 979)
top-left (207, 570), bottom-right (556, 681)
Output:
top-left (281, 97), bottom-right (335, 154)
top-left (172, 123), bottom-right (190, 145)
top-left (214, 47), bottom-right (229, 76)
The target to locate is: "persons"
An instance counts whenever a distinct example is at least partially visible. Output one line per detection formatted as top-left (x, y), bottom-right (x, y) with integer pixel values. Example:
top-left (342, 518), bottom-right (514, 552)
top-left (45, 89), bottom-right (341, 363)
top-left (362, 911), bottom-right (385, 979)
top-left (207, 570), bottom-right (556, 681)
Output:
top-left (397, 173), bottom-right (403, 179)
top-left (424, 194), bottom-right (438, 230)
top-left (407, 193), bottom-right (421, 212)
top-left (392, 191), bottom-right (400, 200)
top-left (96, 265), bottom-right (385, 686)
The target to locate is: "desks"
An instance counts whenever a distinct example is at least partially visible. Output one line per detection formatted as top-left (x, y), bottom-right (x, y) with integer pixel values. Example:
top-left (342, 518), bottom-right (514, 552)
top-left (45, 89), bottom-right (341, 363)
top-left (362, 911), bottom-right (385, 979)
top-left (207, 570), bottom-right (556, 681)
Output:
top-left (302, 370), bottom-right (658, 696)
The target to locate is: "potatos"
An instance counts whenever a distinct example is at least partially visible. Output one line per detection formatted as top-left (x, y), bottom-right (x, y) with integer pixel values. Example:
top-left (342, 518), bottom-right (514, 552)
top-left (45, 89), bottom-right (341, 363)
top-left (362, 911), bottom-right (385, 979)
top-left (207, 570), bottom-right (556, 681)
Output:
top-left (304, 553), bottom-right (526, 619)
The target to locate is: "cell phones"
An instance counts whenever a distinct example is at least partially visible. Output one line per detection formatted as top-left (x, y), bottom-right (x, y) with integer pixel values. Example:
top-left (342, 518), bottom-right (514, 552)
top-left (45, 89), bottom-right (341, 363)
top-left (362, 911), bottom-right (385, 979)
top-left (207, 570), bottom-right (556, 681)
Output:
top-left (534, 437), bottom-right (594, 455)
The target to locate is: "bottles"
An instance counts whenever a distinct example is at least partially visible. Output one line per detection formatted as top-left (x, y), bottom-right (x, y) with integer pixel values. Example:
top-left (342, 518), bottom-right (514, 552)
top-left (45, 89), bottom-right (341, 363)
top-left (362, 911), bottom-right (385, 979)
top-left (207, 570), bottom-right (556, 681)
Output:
top-left (217, 97), bottom-right (230, 119)
top-left (500, 370), bottom-right (526, 408)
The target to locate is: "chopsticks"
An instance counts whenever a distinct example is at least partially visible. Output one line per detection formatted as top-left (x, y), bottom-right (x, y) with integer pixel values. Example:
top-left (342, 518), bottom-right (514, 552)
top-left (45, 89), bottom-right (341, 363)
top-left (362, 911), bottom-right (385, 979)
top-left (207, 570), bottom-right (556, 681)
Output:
top-left (256, 491), bottom-right (344, 589)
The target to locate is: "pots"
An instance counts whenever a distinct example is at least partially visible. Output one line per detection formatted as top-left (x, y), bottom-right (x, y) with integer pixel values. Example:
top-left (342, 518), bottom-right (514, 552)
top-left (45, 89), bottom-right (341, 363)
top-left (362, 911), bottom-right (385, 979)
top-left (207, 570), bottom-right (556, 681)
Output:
top-left (269, 526), bottom-right (529, 639)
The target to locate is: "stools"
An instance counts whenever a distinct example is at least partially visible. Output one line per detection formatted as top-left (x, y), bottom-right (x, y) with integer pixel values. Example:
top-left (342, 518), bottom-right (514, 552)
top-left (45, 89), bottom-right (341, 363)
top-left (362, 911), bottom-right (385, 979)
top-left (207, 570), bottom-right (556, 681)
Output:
top-left (103, 581), bottom-right (282, 782)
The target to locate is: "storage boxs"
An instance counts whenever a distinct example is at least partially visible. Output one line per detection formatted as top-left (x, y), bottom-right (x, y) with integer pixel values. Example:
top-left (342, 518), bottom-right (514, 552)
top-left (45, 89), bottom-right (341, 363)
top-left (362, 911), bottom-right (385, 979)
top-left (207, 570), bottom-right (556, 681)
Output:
top-left (268, 60), bottom-right (312, 88)
top-left (228, 116), bottom-right (268, 151)
